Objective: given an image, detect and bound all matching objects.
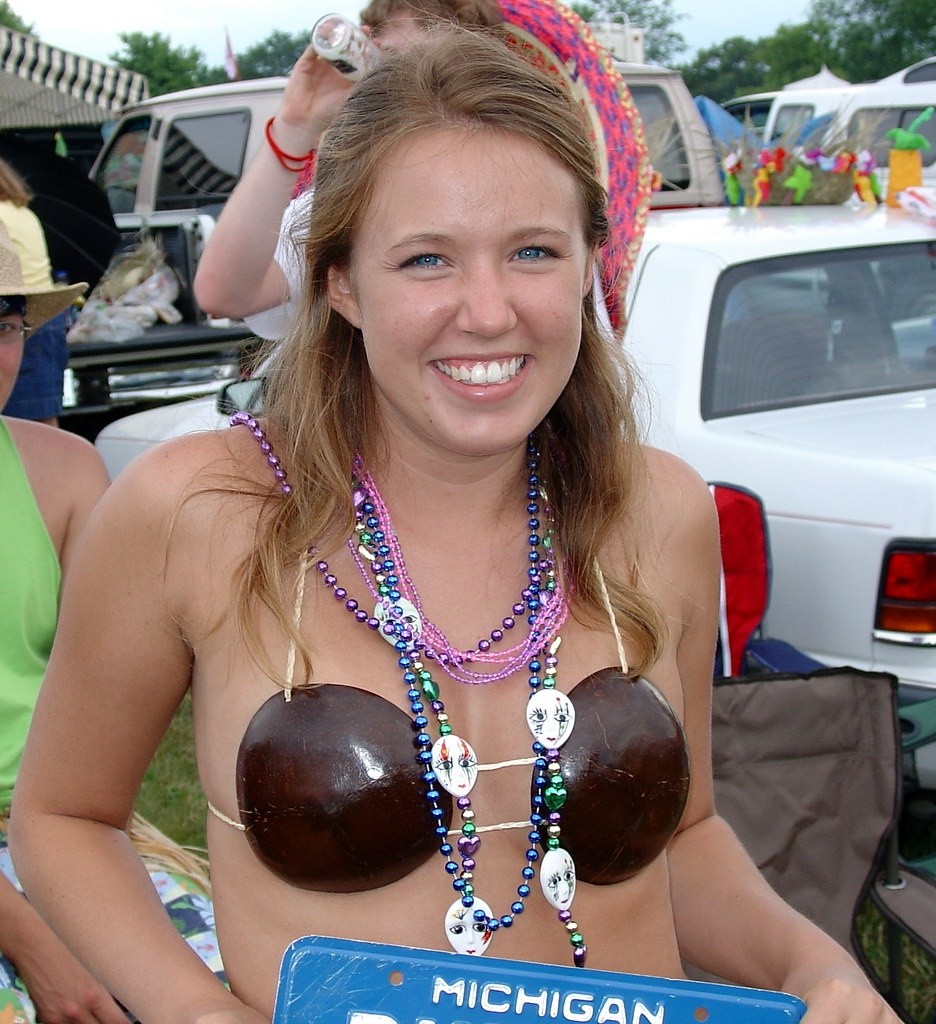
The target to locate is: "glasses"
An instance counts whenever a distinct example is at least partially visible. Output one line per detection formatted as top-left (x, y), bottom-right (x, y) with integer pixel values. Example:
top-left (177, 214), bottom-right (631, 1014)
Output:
top-left (0, 317), bottom-right (32, 343)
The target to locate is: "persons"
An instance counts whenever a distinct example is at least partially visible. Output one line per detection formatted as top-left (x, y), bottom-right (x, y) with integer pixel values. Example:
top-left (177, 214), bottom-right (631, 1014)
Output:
top-left (0, 160), bottom-right (68, 429)
top-left (0, 220), bottom-right (233, 1024)
top-left (7, 24), bottom-right (913, 1024)
top-left (190, 1), bottom-right (508, 345)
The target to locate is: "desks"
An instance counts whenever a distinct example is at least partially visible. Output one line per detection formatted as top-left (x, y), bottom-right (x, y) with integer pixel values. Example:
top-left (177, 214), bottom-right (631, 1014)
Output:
top-left (58, 322), bottom-right (279, 442)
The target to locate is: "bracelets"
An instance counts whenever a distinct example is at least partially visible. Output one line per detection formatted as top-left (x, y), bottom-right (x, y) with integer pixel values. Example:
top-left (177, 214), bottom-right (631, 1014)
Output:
top-left (264, 116), bottom-right (312, 172)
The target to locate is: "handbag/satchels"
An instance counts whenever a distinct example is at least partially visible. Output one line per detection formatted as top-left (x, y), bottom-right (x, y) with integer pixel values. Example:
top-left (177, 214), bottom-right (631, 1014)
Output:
top-left (63, 242), bottom-right (183, 341)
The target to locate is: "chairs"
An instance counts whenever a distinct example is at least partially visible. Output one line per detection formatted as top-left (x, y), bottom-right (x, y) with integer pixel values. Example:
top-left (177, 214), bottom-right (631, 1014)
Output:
top-left (681, 483), bottom-right (936, 1024)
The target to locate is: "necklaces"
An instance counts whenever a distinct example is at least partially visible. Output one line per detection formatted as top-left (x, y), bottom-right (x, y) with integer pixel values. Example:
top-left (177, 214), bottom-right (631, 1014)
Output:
top-left (227, 412), bottom-right (585, 968)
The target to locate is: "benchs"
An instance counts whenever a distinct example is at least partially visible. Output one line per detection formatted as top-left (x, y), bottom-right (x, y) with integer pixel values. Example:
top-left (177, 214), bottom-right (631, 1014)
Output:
top-left (718, 309), bottom-right (935, 411)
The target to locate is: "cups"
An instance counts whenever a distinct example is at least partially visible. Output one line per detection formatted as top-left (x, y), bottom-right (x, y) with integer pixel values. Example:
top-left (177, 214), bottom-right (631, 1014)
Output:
top-left (310, 12), bottom-right (382, 81)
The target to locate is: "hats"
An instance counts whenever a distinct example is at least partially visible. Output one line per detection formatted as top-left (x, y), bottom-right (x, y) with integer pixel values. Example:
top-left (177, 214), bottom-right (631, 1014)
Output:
top-left (0, 198), bottom-right (89, 337)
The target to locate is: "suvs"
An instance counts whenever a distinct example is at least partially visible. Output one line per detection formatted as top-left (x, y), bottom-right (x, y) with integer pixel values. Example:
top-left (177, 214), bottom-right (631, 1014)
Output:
top-left (32, 57), bottom-right (936, 441)
top-left (95, 207), bottom-right (936, 795)
